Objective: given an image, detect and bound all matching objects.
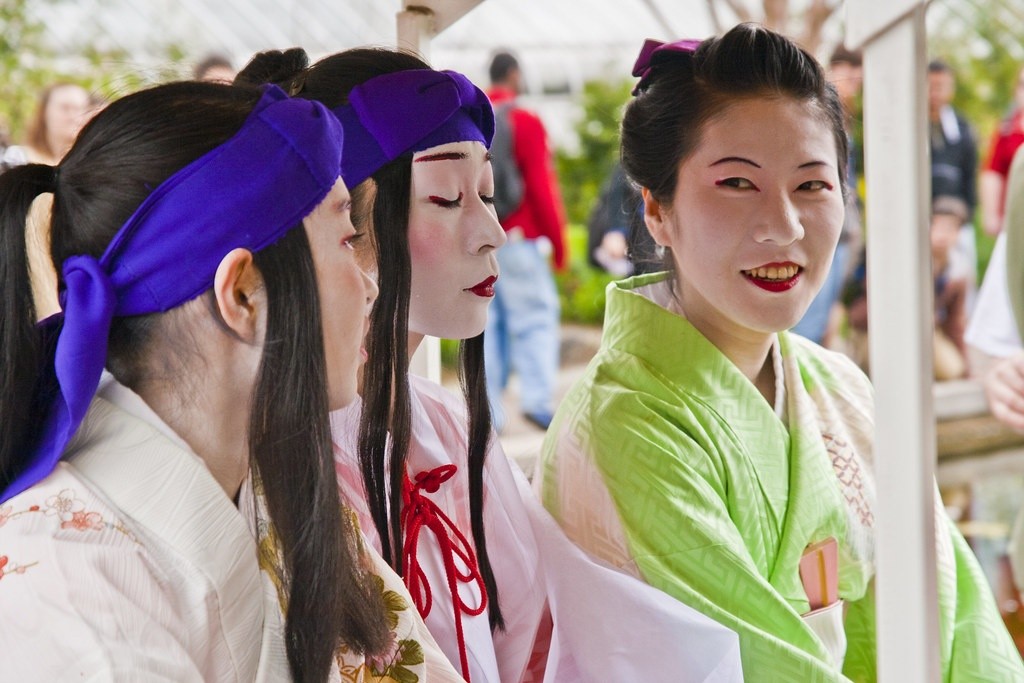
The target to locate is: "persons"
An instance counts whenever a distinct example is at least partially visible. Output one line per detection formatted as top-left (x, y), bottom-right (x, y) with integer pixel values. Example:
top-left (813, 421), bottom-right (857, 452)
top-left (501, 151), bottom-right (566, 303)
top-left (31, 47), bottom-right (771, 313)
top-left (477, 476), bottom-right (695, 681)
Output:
top-left (230, 48), bottom-right (745, 683)
top-left (484, 53), bottom-right (571, 435)
top-left (588, 49), bottom-right (1024, 431)
top-left (0, 53), bottom-right (238, 166)
top-left (531, 22), bottom-right (1024, 683)
top-left (0, 79), bottom-right (466, 683)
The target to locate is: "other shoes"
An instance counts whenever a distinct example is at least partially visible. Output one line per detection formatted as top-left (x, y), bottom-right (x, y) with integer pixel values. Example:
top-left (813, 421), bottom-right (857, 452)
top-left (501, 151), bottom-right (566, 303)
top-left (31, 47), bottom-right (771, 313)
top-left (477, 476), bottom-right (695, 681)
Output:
top-left (524, 408), bottom-right (558, 427)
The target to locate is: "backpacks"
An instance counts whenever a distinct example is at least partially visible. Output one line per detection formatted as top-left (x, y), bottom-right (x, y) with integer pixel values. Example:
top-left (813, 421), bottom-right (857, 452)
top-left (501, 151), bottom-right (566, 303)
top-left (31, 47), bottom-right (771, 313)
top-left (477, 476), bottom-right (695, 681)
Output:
top-left (487, 102), bottom-right (523, 218)
top-left (587, 177), bottom-right (646, 283)
